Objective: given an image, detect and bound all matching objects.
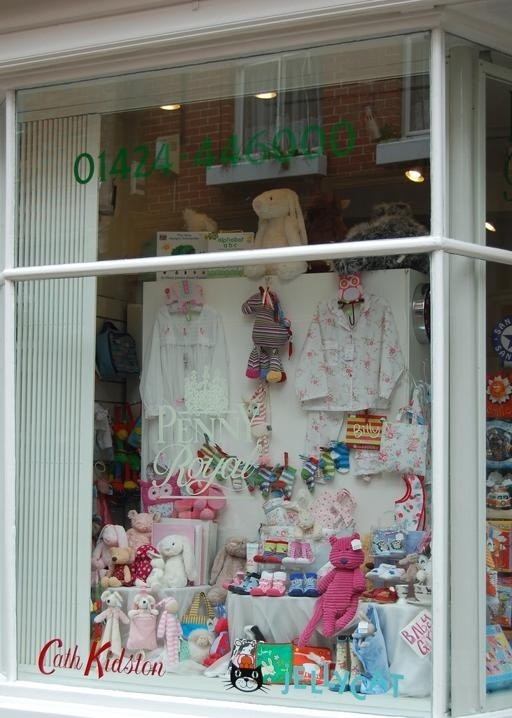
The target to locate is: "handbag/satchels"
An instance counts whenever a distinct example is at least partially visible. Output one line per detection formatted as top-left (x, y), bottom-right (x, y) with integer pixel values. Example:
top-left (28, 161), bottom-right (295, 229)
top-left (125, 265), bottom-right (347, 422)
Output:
top-left (379, 408), bottom-right (428, 476)
top-left (180, 592), bottom-right (214, 660)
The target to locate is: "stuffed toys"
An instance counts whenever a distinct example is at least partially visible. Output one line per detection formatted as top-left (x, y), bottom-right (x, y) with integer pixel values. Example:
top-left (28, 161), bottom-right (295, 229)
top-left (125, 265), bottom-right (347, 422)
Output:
top-left (91, 187), bottom-right (434, 692)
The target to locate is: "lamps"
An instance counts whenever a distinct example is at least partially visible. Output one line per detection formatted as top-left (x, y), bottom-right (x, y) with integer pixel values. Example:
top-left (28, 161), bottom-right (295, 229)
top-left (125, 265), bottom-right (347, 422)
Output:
top-left (405, 165), bottom-right (425, 183)
top-left (485, 218), bottom-right (497, 231)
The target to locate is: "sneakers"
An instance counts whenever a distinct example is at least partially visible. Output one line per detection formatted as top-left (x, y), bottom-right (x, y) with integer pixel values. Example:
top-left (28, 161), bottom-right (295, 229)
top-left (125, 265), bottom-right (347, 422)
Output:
top-left (362, 535), bottom-right (405, 603)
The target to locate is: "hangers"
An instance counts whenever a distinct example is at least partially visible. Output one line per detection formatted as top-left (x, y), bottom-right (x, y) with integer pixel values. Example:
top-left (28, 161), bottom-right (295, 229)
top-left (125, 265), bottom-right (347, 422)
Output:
top-left (168, 302), bottom-right (202, 313)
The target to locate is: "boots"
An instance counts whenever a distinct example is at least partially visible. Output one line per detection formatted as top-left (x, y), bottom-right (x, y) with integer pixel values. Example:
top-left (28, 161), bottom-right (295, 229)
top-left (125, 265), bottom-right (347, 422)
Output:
top-left (329, 636), bottom-right (349, 691)
top-left (253, 540), bottom-right (315, 566)
top-left (224, 572), bottom-right (319, 597)
top-left (348, 637), bottom-right (363, 691)
